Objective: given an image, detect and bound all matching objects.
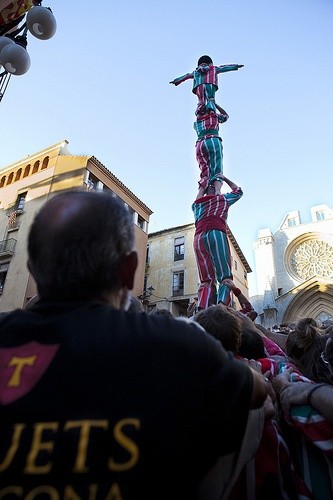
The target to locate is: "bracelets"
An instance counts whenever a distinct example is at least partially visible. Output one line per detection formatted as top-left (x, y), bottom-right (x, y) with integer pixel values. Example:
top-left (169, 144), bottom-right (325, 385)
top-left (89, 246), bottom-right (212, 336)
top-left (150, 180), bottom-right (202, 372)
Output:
top-left (233, 288), bottom-right (242, 297)
top-left (305, 383), bottom-right (328, 404)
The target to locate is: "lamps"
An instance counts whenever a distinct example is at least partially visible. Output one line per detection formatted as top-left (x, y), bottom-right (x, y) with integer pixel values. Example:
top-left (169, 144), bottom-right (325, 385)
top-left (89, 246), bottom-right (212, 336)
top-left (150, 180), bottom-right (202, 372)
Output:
top-left (0, 5), bottom-right (57, 101)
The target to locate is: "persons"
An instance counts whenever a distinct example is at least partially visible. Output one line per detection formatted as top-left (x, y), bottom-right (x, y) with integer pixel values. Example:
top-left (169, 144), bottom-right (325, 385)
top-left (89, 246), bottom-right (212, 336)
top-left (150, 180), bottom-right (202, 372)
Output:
top-left (193, 103), bottom-right (229, 201)
top-left (191, 174), bottom-right (243, 318)
top-left (0, 186), bottom-right (269, 500)
top-left (169, 55), bottom-right (244, 119)
top-left (128, 279), bottom-right (333, 500)
